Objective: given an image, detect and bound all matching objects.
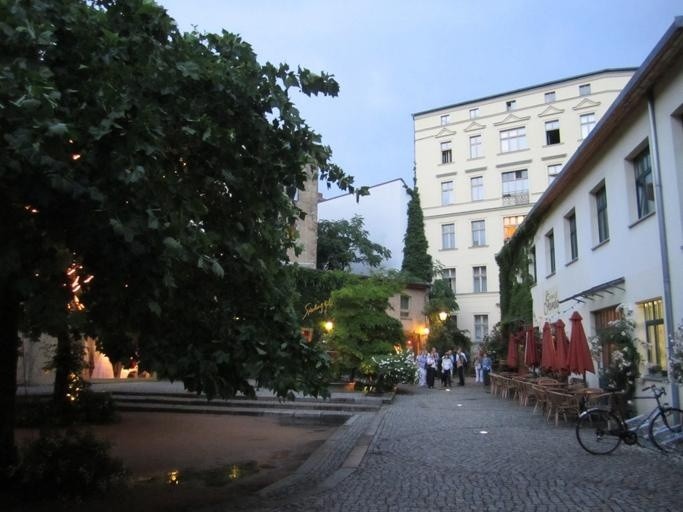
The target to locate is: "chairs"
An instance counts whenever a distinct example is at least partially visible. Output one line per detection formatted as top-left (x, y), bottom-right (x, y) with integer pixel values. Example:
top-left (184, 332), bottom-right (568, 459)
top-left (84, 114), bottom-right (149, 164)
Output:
top-left (487, 371), bottom-right (626, 427)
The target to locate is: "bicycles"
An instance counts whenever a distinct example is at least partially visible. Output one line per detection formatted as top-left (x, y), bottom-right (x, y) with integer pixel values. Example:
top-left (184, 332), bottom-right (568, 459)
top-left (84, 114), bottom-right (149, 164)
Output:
top-left (572, 382), bottom-right (681, 458)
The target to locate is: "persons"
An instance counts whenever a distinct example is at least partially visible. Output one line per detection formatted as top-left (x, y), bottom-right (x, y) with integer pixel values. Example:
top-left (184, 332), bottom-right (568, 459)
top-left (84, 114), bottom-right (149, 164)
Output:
top-left (417, 346), bottom-right (493, 389)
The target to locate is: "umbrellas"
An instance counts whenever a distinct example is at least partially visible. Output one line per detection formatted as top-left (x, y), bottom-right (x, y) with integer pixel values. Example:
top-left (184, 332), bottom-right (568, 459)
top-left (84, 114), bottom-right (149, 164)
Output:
top-left (552, 318), bottom-right (572, 387)
top-left (566, 310), bottom-right (596, 384)
top-left (539, 320), bottom-right (556, 377)
top-left (507, 331), bottom-right (519, 373)
top-left (525, 324), bottom-right (540, 377)
